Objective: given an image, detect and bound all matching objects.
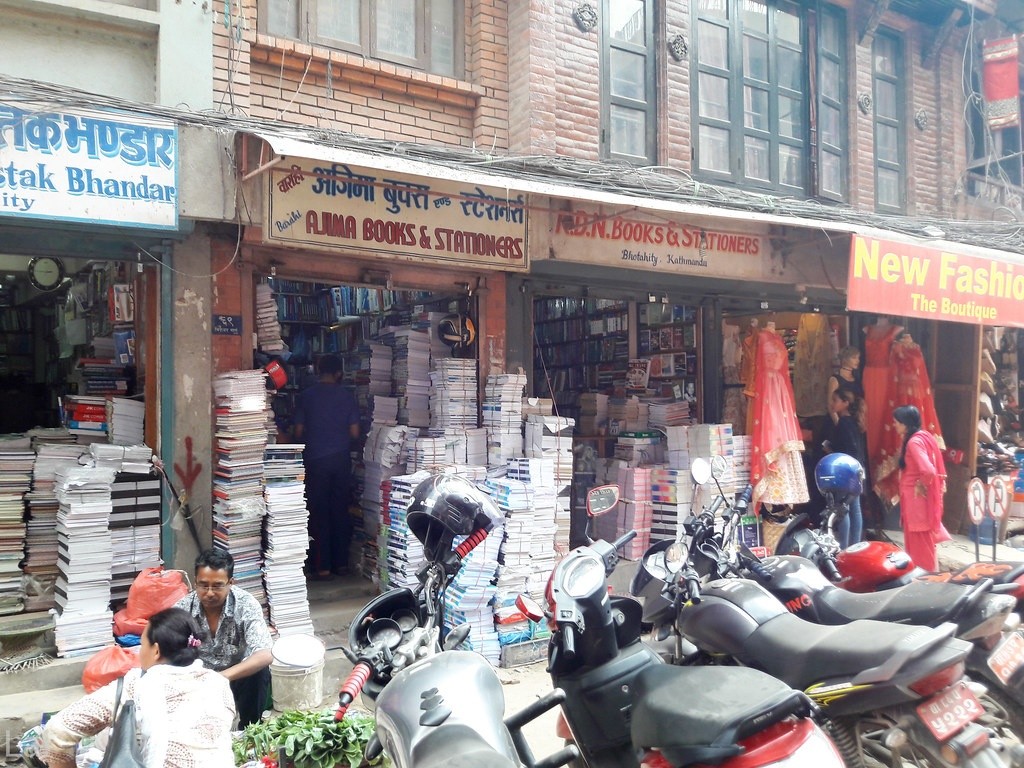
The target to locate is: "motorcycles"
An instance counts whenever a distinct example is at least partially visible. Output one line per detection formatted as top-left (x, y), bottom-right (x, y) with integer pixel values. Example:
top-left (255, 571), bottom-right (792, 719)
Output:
top-left (514, 484), bottom-right (861, 768)
top-left (267, 517), bottom-right (578, 768)
top-left (631, 453), bottom-right (1024, 768)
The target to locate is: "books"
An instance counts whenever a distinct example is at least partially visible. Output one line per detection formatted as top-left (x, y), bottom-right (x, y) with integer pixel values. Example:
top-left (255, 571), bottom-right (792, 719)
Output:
top-left (255, 277), bottom-right (576, 669)
top-left (0, 265), bottom-right (161, 658)
top-left (535, 298), bottom-right (765, 562)
top-left (213, 370), bottom-right (315, 638)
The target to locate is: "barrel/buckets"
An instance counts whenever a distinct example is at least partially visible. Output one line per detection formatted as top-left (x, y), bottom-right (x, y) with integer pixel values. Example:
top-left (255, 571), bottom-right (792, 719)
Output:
top-left (268, 633), bottom-right (326, 712)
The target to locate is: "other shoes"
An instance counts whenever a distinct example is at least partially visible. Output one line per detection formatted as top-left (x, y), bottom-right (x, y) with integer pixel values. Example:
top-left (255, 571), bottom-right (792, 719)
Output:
top-left (337, 568), bottom-right (348, 575)
top-left (976, 332), bottom-right (1024, 475)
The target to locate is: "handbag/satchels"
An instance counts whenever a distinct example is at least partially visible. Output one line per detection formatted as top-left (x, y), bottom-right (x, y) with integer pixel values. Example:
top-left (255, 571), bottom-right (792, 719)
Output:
top-left (98, 677), bottom-right (145, 768)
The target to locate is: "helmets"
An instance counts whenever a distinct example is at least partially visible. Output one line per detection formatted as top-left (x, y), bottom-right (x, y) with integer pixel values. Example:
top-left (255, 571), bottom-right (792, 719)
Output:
top-left (815, 453), bottom-right (863, 496)
top-left (406, 474), bottom-right (484, 562)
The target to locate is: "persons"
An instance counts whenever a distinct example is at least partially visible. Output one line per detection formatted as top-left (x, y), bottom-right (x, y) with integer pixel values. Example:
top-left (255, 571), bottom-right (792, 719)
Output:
top-left (39, 607), bottom-right (236, 768)
top-left (827, 316), bottom-right (946, 512)
top-left (893, 405), bottom-right (947, 573)
top-left (721, 318), bottom-right (810, 517)
top-left (171, 548), bottom-right (274, 730)
top-left (822, 389), bottom-right (865, 548)
top-left (293, 354), bottom-right (360, 582)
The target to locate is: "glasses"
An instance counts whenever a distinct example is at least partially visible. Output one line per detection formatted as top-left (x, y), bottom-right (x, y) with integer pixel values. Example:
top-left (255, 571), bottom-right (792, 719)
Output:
top-left (195, 580), bottom-right (229, 592)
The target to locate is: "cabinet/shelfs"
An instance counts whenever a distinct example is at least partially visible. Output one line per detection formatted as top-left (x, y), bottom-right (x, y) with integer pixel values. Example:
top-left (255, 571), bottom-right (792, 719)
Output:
top-left (261, 281), bottom-right (475, 446)
top-left (531, 295), bottom-right (701, 553)
